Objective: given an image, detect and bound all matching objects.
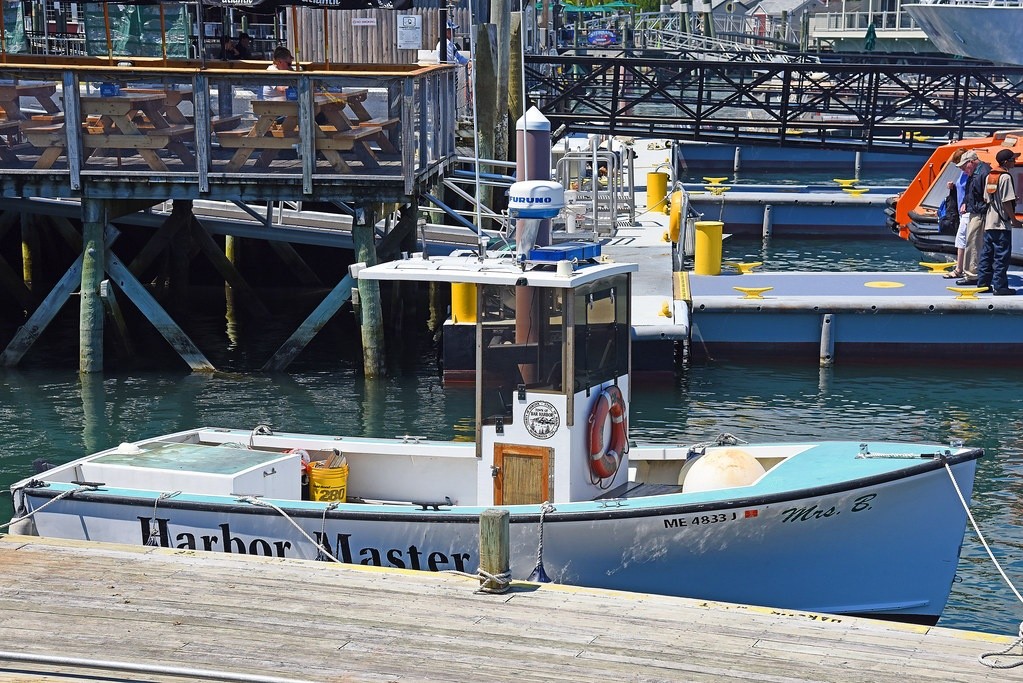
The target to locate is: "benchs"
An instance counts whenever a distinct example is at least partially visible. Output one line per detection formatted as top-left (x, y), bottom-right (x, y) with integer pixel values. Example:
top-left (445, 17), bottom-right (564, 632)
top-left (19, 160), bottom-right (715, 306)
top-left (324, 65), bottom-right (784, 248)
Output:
top-left (210, 114), bottom-right (242, 125)
top-left (0, 120), bottom-right (20, 130)
top-left (360, 117), bottom-right (399, 127)
top-left (217, 125), bottom-right (274, 137)
top-left (332, 126), bottom-right (382, 140)
top-left (22, 122), bottom-right (89, 135)
top-left (31, 112), bottom-right (64, 120)
top-left (146, 125), bottom-right (195, 136)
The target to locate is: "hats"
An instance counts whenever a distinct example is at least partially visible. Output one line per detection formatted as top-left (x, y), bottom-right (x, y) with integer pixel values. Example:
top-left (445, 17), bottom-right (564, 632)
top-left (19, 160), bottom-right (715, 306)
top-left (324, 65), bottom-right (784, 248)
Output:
top-left (239, 33), bottom-right (253, 40)
top-left (996, 149), bottom-right (1021, 163)
top-left (955, 151), bottom-right (978, 166)
top-left (446, 20), bottom-right (460, 29)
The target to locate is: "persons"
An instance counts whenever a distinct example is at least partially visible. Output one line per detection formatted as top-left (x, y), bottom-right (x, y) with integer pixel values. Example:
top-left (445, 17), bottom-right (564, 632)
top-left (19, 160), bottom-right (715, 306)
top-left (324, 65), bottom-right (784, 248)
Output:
top-left (977, 149), bottom-right (1023, 296)
top-left (261, 45), bottom-right (297, 138)
top-left (942, 148), bottom-right (966, 278)
top-left (220, 35), bottom-right (244, 60)
top-left (955, 149), bottom-right (991, 285)
top-left (235, 32), bottom-right (254, 60)
top-left (436, 20), bottom-right (473, 73)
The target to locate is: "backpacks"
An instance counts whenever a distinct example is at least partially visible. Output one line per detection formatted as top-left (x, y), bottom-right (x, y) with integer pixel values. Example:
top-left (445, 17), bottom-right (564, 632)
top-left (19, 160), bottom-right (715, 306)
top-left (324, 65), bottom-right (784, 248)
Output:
top-left (938, 185), bottom-right (959, 236)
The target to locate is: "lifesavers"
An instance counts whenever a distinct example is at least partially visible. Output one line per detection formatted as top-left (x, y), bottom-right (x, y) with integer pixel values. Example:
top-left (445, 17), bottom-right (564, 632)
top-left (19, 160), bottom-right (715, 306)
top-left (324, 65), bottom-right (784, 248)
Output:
top-left (584, 384), bottom-right (627, 478)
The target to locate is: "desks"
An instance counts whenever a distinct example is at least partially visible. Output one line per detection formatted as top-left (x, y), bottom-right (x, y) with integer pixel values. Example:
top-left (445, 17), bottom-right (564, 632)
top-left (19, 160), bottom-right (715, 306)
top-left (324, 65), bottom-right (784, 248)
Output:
top-left (0, 84), bottom-right (45, 169)
top-left (222, 95), bottom-right (380, 174)
top-left (90, 87), bottom-right (221, 155)
top-left (272, 89), bottom-right (397, 161)
top-left (31, 93), bottom-right (196, 172)
top-left (0, 81), bottom-right (62, 145)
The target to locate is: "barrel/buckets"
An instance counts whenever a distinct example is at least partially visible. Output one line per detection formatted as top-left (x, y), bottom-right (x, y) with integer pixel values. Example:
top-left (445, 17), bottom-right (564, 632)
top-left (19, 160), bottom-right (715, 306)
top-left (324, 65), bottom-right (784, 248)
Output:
top-left (300, 466), bottom-right (309, 500)
top-left (306, 460), bottom-right (349, 503)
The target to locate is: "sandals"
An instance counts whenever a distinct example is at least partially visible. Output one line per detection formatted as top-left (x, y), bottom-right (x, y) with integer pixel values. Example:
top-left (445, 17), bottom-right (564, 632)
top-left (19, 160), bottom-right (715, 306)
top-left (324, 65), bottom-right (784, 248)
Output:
top-left (943, 270), bottom-right (963, 279)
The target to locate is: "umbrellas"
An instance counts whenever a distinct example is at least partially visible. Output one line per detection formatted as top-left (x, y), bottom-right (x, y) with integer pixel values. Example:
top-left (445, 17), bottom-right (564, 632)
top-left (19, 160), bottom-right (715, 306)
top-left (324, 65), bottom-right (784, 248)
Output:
top-left (0, 0), bottom-right (414, 66)
top-left (865, 24), bottom-right (877, 51)
top-left (534, 0), bottom-right (641, 28)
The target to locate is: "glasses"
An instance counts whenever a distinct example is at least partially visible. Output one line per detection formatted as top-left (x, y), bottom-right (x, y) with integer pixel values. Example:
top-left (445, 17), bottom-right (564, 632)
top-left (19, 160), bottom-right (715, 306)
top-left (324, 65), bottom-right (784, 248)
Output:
top-left (286, 61), bottom-right (291, 66)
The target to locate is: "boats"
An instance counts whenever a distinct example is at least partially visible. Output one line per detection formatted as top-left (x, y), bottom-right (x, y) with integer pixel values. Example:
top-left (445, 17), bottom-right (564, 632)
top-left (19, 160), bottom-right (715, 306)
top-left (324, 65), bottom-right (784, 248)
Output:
top-left (10, 106), bottom-right (985, 625)
top-left (883, 130), bottom-right (1023, 264)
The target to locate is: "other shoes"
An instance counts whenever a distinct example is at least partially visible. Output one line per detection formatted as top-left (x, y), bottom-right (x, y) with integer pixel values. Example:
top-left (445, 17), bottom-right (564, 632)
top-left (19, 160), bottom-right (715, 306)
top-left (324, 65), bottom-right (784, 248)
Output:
top-left (994, 288), bottom-right (1016, 295)
top-left (978, 286), bottom-right (993, 293)
top-left (956, 279), bottom-right (978, 285)
